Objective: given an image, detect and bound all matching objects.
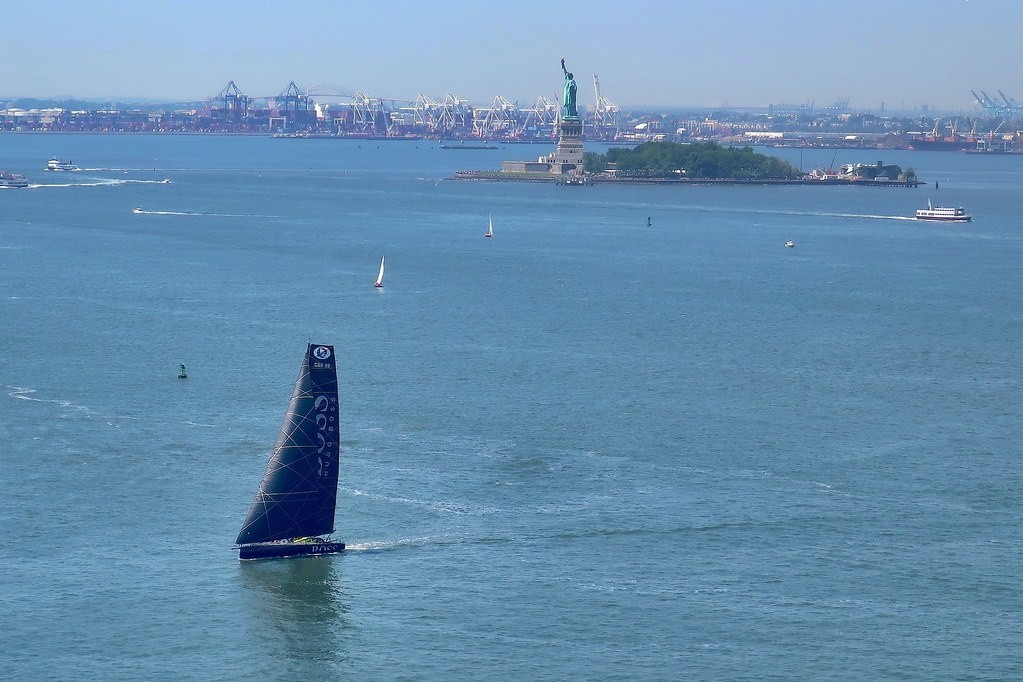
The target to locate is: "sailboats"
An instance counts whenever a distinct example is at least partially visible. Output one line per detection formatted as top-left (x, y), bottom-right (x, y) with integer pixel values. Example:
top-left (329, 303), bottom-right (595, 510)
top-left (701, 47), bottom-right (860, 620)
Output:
top-left (374, 253), bottom-right (385, 287)
top-left (485, 212), bottom-right (493, 237)
top-left (230, 343), bottom-right (345, 561)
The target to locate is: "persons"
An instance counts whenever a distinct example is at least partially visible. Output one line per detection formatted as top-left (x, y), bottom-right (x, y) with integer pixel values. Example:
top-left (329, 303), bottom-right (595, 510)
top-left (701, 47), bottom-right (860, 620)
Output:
top-left (561, 61), bottom-right (578, 116)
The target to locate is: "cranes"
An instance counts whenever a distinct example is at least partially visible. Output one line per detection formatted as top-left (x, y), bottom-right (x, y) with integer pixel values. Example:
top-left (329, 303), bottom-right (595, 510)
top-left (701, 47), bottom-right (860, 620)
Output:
top-left (971, 89), bottom-right (1019, 120)
top-left (338, 91), bottom-right (562, 134)
top-left (591, 74), bottom-right (622, 127)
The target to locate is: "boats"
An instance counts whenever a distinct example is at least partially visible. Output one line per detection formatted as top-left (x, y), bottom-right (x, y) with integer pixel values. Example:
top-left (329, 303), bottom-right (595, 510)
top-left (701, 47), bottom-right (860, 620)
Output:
top-left (43, 156), bottom-right (78, 173)
top-left (916, 198), bottom-right (972, 223)
top-left (785, 240), bottom-right (794, 247)
top-left (0, 170), bottom-right (28, 187)
